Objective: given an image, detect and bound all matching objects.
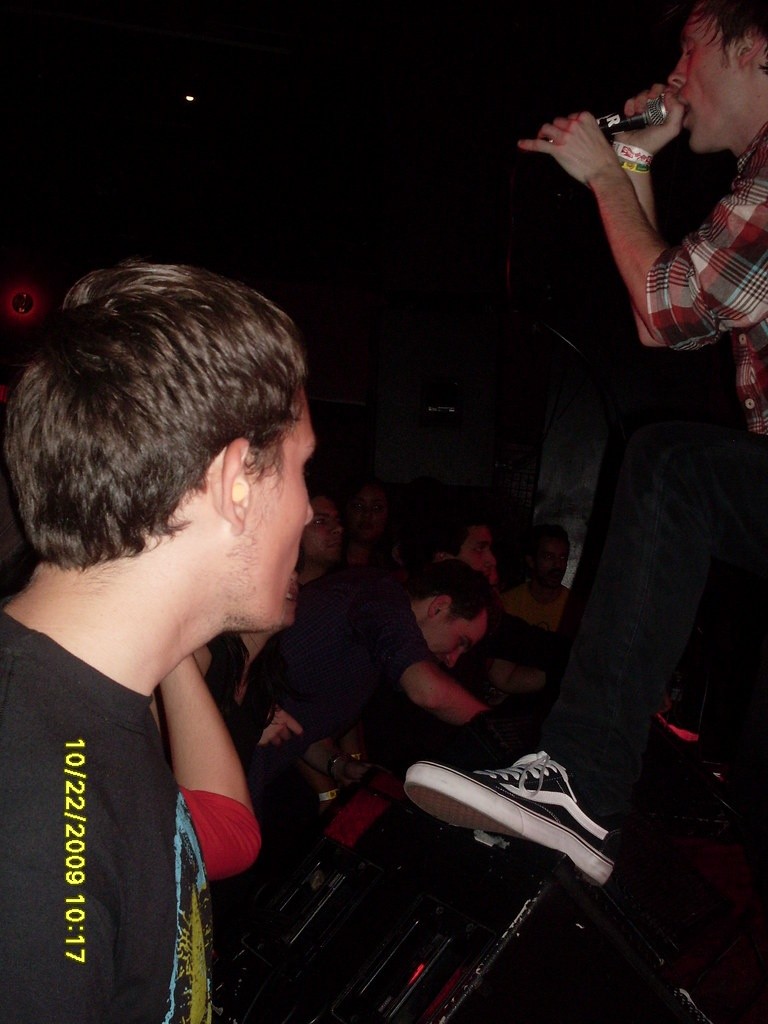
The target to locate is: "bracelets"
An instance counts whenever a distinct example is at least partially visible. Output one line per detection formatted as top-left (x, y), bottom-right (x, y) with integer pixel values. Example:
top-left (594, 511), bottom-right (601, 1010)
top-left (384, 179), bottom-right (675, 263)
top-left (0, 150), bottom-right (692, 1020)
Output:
top-left (319, 789), bottom-right (339, 801)
top-left (328, 753), bottom-right (350, 779)
top-left (612, 142), bottom-right (652, 175)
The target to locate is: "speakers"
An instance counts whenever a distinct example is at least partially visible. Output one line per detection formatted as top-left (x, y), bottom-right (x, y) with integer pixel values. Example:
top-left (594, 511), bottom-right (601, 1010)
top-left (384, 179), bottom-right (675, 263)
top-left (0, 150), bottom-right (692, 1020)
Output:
top-left (211, 767), bottom-right (714, 1024)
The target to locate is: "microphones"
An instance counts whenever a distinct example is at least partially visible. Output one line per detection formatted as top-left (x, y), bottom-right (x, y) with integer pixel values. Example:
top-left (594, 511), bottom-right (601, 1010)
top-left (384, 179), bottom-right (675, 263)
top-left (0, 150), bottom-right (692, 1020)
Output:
top-left (547, 92), bottom-right (684, 144)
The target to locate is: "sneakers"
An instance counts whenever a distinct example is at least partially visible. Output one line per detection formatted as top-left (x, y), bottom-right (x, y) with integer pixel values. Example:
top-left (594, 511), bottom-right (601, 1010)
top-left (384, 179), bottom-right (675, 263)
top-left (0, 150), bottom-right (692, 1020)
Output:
top-left (404, 751), bottom-right (620, 888)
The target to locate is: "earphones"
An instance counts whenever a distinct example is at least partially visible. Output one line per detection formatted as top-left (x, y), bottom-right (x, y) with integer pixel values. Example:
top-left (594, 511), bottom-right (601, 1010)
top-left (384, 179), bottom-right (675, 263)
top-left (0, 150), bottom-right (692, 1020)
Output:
top-left (232, 484), bottom-right (246, 502)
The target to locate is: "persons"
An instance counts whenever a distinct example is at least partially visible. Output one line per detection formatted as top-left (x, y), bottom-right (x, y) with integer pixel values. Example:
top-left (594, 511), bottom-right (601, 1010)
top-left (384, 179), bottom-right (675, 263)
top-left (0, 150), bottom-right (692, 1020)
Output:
top-left (195, 465), bottom-right (588, 822)
top-left (0, 259), bottom-right (317, 1024)
top-left (403, 0), bottom-right (768, 887)
